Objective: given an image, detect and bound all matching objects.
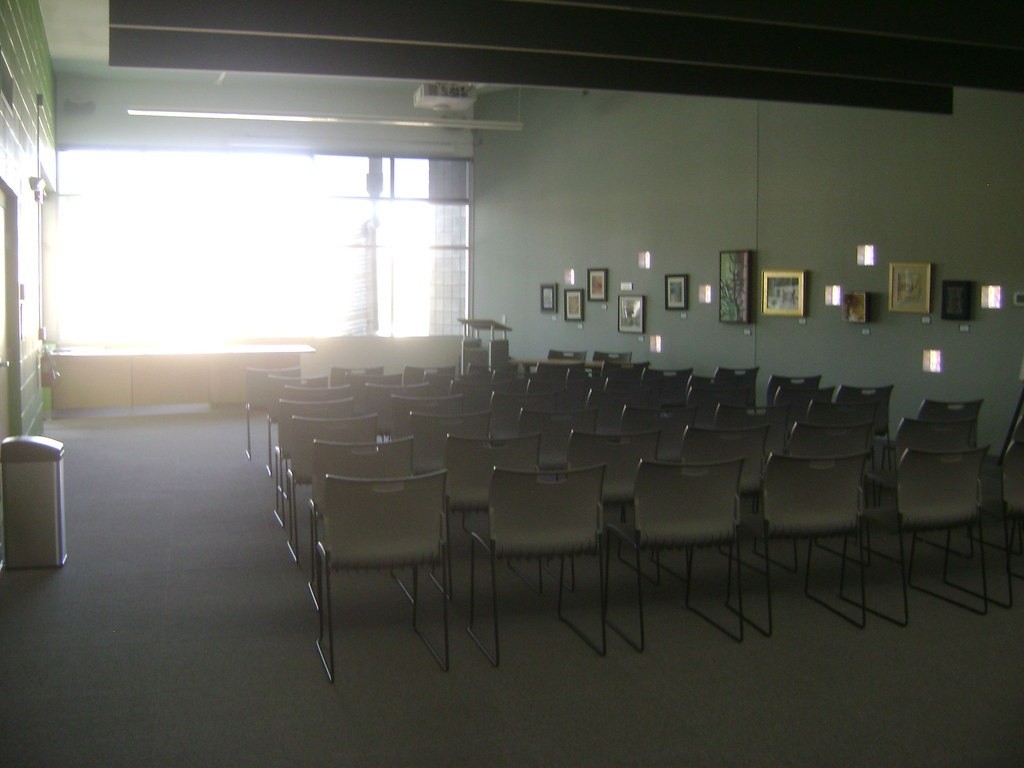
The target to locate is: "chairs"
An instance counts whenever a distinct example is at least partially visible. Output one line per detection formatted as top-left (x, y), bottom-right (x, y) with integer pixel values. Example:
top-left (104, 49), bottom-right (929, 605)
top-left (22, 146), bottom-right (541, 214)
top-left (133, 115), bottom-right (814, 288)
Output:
top-left (244, 348), bottom-right (1024, 683)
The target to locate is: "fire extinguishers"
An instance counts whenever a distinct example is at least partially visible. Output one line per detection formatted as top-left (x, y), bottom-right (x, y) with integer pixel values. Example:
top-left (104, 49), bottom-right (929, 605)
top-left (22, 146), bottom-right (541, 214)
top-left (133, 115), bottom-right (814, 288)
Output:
top-left (40, 352), bottom-right (60, 387)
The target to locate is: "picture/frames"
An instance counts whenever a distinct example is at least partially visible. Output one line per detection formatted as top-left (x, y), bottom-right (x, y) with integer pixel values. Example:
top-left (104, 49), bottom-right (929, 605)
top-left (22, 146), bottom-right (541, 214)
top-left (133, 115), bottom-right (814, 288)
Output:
top-left (719, 249), bottom-right (750, 321)
top-left (761, 267), bottom-right (806, 316)
top-left (617, 294), bottom-right (644, 333)
top-left (887, 261), bottom-right (933, 313)
top-left (564, 288), bottom-right (584, 320)
top-left (664, 274), bottom-right (688, 309)
top-left (540, 282), bottom-right (557, 313)
top-left (841, 292), bottom-right (867, 322)
top-left (586, 268), bottom-right (607, 301)
top-left (940, 279), bottom-right (971, 321)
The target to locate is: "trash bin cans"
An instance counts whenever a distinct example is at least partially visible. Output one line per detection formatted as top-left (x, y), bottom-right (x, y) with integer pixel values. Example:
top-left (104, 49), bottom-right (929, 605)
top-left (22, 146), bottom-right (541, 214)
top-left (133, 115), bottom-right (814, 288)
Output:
top-left (0, 435), bottom-right (68, 569)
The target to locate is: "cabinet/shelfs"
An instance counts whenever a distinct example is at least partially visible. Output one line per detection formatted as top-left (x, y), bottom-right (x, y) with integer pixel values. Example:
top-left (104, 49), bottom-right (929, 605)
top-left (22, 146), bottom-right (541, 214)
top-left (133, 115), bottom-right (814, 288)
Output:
top-left (51, 344), bottom-right (316, 419)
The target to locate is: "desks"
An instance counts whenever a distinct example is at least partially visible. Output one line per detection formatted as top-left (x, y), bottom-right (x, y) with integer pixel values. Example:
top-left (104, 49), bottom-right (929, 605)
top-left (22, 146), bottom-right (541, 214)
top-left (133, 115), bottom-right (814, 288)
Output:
top-left (512, 359), bottom-right (637, 376)
